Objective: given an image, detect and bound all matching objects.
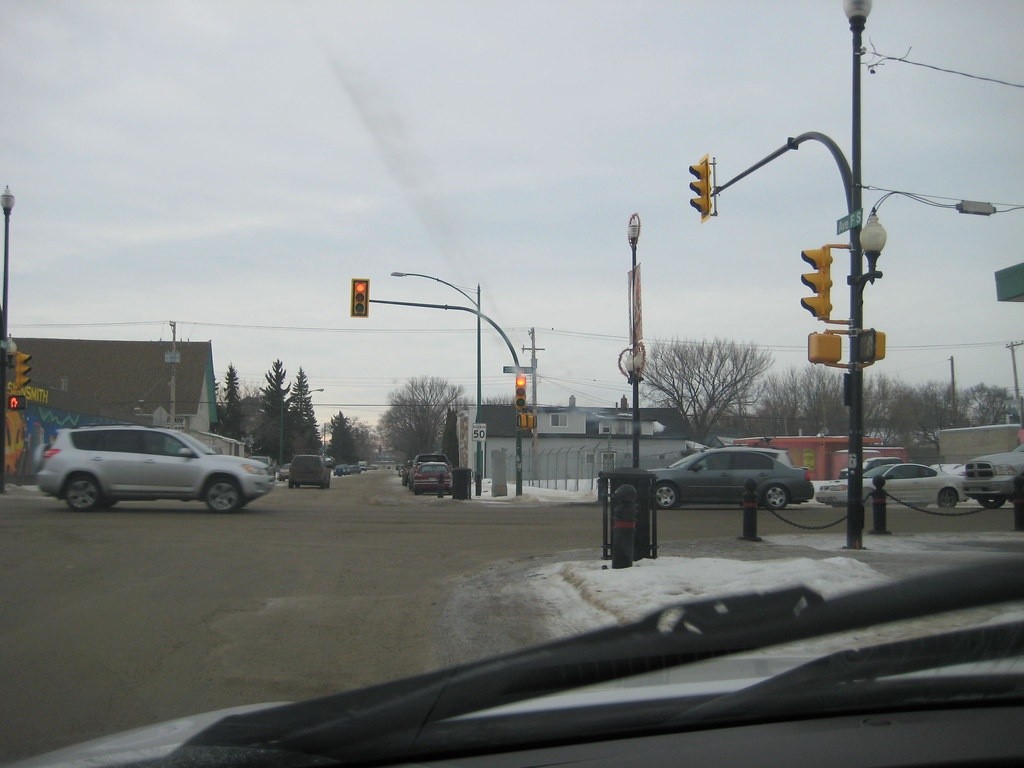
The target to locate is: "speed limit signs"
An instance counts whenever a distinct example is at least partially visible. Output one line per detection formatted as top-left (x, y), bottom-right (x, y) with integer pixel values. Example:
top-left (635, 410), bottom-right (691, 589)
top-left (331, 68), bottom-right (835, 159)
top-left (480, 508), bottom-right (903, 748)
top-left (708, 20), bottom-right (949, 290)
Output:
top-left (472, 423), bottom-right (487, 442)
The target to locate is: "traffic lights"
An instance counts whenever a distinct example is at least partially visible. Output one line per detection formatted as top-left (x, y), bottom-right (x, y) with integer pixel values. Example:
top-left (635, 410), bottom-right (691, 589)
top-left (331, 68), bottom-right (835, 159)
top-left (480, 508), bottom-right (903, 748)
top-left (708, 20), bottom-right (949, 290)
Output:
top-left (16, 352), bottom-right (32, 389)
top-left (350, 278), bottom-right (369, 318)
top-left (856, 327), bottom-right (886, 361)
top-left (518, 415), bottom-right (537, 429)
top-left (689, 153), bottom-right (713, 223)
top-left (801, 247), bottom-right (830, 322)
top-left (7, 395), bottom-right (27, 410)
top-left (516, 376), bottom-right (526, 408)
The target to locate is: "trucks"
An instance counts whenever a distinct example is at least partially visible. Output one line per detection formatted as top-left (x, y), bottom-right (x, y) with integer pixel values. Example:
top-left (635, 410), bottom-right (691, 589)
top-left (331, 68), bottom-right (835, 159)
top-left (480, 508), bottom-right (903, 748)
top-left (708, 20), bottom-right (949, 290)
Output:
top-left (359, 461), bottom-right (367, 471)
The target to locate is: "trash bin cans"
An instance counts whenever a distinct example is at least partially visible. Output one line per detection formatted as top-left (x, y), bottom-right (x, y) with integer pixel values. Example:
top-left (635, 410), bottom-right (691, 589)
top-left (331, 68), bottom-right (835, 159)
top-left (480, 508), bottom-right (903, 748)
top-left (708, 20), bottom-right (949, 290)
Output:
top-left (452, 467), bottom-right (472, 499)
top-left (597, 467), bottom-right (659, 560)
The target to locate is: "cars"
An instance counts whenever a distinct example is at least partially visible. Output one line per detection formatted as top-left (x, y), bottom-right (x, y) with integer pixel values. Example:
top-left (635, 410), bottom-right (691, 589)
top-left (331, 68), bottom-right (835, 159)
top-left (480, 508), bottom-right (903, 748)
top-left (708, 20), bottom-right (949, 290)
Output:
top-left (398, 460), bottom-right (413, 486)
top-left (667, 447), bottom-right (794, 469)
top-left (333, 464), bottom-right (361, 477)
top-left (413, 462), bottom-right (453, 495)
top-left (815, 463), bottom-right (969, 509)
top-left (646, 447), bottom-right (814, 510)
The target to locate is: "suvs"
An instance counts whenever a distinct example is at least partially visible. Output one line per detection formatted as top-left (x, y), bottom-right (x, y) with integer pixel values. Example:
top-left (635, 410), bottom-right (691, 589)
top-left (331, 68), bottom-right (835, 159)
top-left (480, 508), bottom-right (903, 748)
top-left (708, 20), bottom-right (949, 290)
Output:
top-left (279, 463), bottom-right (291, 481)
top-left (288, 453), bottom-right (331, 489)
top-left (407, 453), bottom-right (452, 491)
top-left (962, 443), bottom-right (1024, 510)
top-left (37, 424), bottom-right (275, 514)
top-left (248, 456), bottom-right (275, 480)
top-left (839, 456), bottom-right (906, 479)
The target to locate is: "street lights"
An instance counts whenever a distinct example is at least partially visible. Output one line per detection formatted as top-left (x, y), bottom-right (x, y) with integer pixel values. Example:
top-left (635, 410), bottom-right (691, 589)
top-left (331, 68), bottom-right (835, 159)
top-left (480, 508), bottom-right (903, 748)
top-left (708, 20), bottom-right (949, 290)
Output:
top-left (391, 272), bottom-right (483, 494)
top-left (624, 214), bottom-right (645, 468)
top-left (0, 184), bottom-right (18, 486)
top-left (844, 1), bottom-right (886, 549)
top-left (279, 388), bottom-right (325, 466)
